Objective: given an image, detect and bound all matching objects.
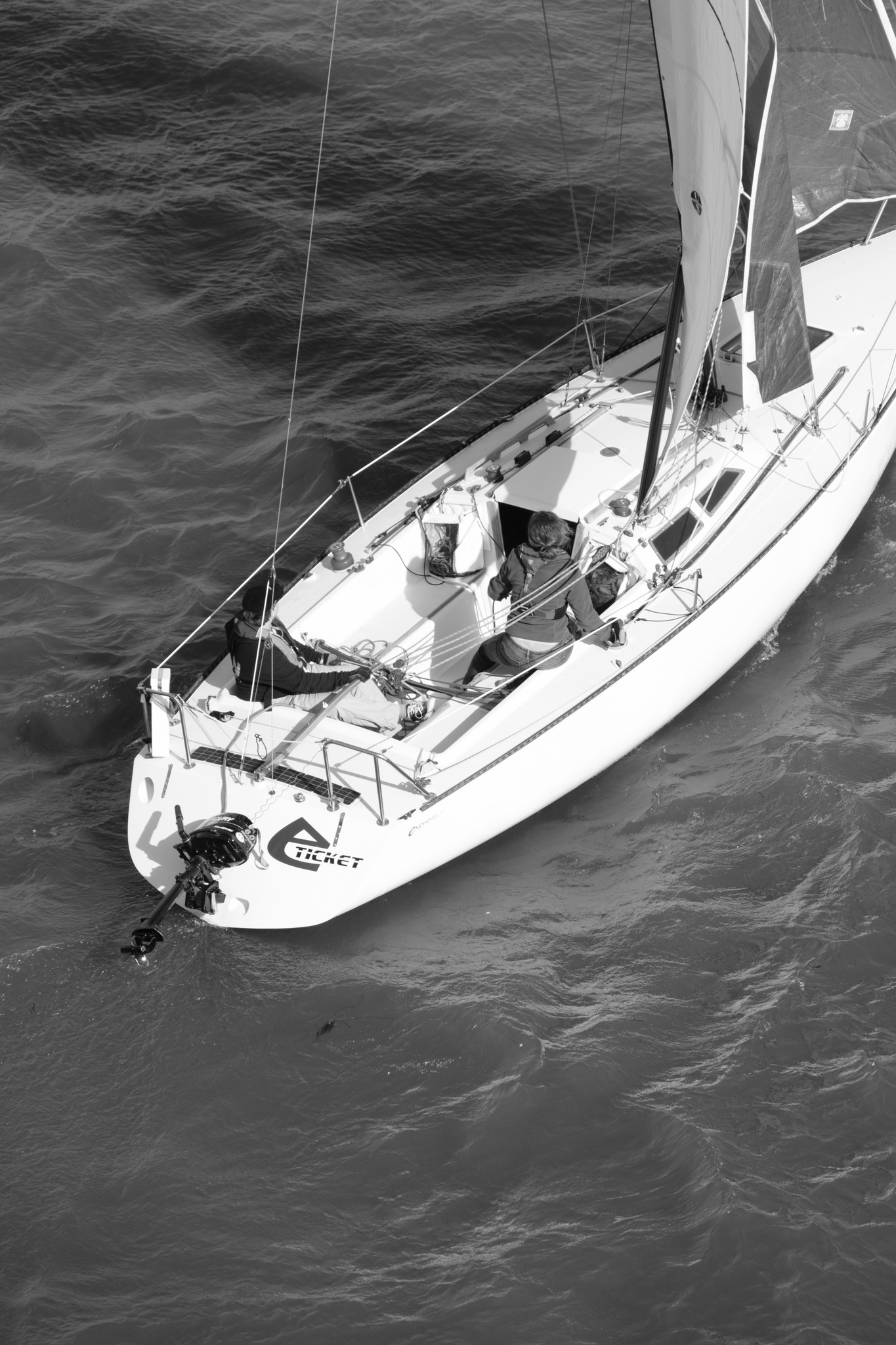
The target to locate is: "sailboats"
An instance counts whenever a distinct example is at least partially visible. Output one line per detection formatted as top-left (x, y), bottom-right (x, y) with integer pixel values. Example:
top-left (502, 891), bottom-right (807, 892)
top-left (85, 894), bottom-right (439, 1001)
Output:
top-left (106, 0), bottom-right (896, 976)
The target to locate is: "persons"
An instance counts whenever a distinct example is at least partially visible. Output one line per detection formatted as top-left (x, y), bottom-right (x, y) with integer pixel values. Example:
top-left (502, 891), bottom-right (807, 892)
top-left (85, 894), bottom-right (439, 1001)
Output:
top-left (224, 583), bottom-right (436, 728)
top-left (458, 510), bottom-right (619, 684)
